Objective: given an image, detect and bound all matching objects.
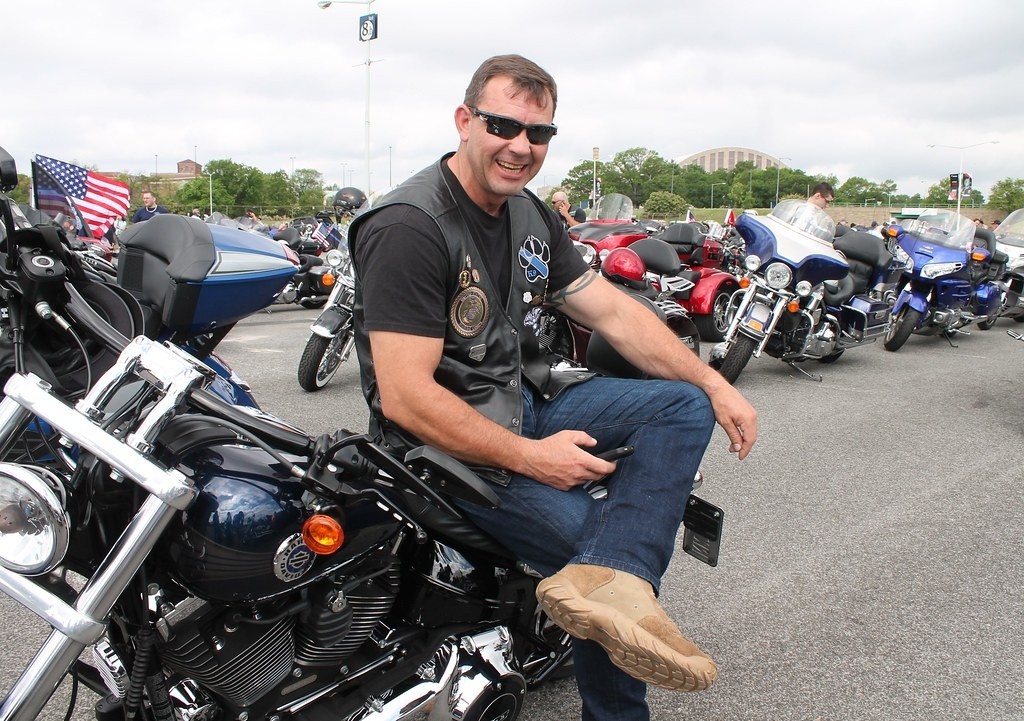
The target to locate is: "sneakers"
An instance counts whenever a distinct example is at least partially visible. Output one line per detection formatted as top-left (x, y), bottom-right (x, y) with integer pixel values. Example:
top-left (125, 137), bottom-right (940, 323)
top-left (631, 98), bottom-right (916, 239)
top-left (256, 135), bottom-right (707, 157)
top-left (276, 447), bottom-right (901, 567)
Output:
top-left (536, 562), bottom-right (717, 692)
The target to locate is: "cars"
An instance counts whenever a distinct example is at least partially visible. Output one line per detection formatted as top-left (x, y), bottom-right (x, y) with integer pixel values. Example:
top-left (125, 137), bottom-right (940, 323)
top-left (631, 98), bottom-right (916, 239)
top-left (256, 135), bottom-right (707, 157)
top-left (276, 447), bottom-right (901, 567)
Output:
top-left (992, 208), bottom-right (1024, 274)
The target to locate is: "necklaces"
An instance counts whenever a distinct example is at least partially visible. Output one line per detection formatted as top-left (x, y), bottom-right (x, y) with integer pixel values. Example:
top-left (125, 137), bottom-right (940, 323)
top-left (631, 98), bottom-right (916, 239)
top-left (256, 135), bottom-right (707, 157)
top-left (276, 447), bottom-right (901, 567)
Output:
top-left (145, 205), bottom-right (157, 213)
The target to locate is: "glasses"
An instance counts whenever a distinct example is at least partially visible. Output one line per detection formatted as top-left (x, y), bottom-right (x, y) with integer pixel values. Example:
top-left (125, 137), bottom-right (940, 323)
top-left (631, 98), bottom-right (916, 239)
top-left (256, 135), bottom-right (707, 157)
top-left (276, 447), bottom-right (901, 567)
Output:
top-left (822, 195), bottom-right (833, 204)
top-left (551, 200), bottom-right (563, 204)
top-left (467, 106), bottom-right (557, 144)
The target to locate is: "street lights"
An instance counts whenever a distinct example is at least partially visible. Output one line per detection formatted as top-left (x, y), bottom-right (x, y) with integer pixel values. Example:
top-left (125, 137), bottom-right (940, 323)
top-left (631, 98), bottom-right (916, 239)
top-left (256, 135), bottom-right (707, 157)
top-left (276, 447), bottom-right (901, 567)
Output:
top-left (290, 156), bottom-right (296, 181)
top-left (195, 145), bottom-right (198, 175)
top-left (340, 162), bottom-right (348, 190)
top-left (155, 154), bottom-right (158, 178)
top-left (711, 183), bottom-right (727, 209)
top-left (776, 158), bottom-right (792, 206)
top-left (346, 168), bottom-right (353, 189)
top-left (205, 171), bottom-right (216, 217)
top-left (865, 198), bottom-right (875, 207)
top-left (318, 0), bottom-right (381, 198)
top-left (393, 145), bottom-right (393, 193)
top-left (927, 138), bottom-right (1000, 237)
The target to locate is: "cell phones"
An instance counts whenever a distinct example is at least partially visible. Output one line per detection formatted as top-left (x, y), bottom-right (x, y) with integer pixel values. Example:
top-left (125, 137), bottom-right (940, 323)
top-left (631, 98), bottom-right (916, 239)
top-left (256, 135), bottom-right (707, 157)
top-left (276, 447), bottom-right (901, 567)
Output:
top-left (596, 445), bottom-right (635, 461)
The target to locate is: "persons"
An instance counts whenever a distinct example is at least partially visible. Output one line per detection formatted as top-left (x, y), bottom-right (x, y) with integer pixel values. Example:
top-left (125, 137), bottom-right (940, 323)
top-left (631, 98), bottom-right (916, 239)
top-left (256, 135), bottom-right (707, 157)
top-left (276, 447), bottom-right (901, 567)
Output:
top-left (790, 182), bottom-right (834, 236)
top-left (850, 220), bottom-right (896, 233)
top-left (836, 216), bottom-right (848, 227)
top-left (191, 209), bottom-right (201, 221)
top-left (245, 211), bottom-right (287, 238)
top-left (132, 191), bottom-right (170, 224)
top-left (553, 191), bottom-right (586, 230)
top-left (974, 218), bottom-right (1005, 238)
top-left (78, 218), bottom-right (127, 251)
top-left (348, 54), bottom-right (760, 721)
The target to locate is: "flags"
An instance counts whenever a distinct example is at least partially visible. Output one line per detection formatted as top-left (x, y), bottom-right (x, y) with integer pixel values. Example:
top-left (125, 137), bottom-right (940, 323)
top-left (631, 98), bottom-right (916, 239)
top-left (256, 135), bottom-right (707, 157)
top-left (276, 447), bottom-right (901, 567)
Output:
top-left (689, 211), bottom-right (696, 222)
top-left (727, 210), bottom-right (736, 226)
top-left (315, 225), bottom-right (330, 247)
top-left (36, 154), bottom-right (131, 238)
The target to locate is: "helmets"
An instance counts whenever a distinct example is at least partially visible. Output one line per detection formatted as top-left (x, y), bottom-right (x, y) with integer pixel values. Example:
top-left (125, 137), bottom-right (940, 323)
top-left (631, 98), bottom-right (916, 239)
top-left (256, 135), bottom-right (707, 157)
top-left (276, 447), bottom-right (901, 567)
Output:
top-left (333, 187), bottom-right (369, 210)
top-left (601, 248), bottom-right (648, 290)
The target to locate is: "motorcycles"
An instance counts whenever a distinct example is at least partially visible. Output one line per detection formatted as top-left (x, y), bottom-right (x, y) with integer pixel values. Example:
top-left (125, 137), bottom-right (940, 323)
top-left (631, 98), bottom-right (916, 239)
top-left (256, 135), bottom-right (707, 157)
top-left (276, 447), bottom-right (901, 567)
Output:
top-left (880, 207), bottom-right (1011, 351)
top-left (297, 202), bottom-right (370, 395)
top-left (552, 193), bottom-right (913, 386)
top-left (0, 145), bottom-right (356, 488)
top-left (0, 191), bottom-right (724, 721)
